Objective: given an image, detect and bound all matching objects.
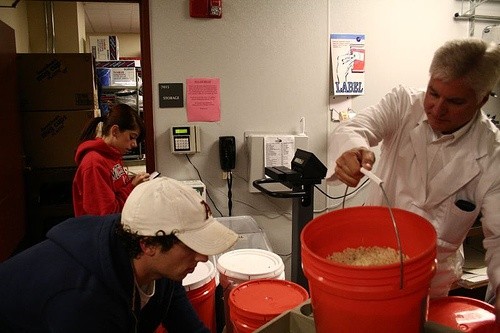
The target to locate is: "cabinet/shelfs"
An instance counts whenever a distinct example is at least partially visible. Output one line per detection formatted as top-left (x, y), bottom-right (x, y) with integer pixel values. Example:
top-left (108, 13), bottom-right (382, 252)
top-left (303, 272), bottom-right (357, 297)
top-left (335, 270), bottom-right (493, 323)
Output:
top-left (96, 60), bottom-right (142, 159)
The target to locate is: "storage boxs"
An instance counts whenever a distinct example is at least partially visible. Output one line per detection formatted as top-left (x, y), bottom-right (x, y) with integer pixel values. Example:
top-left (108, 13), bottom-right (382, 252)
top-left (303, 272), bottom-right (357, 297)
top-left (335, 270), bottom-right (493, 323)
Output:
top-left (18, 109), bottom-right (97, 168)
top-left (17, 51), bottom-right (96, 110)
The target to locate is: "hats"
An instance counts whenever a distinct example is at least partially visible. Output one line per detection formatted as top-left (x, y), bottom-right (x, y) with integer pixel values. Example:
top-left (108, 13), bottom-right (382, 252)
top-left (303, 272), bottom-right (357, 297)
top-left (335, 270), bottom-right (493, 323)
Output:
top-left (121, 177), bottom-right (239, 256)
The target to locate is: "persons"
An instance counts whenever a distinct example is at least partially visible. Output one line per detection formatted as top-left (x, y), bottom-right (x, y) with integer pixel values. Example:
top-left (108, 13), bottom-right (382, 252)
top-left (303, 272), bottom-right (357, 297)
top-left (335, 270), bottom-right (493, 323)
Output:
top-left (0, 176), bottom-right (239, 333)
top-left (71, 103), bottom-right (150, 217)
top-left (323, 38), bottom-right (500, 319)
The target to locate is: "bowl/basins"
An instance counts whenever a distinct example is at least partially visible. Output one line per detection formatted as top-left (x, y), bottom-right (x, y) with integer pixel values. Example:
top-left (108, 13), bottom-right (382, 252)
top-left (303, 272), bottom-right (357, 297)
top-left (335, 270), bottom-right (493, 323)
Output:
top-left (425, 296), bottom-right (500, 333)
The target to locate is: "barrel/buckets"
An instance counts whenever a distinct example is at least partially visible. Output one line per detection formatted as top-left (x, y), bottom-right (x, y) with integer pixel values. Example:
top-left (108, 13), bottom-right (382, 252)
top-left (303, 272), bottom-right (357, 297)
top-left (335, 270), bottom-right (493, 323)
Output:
top-left (300, 167), bottom-right (438, 333)
top-left (227, 278), bottom-right (309, 333)
top-left (216, 248), bottom-right (287, 328)
top-left (155, 259), bottom-right (219, 333)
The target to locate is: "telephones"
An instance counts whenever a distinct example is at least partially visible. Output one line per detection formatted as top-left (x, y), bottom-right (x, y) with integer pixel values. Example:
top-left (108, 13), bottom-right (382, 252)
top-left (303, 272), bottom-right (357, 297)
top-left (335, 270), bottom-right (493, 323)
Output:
top-left (219, 135), bottom-right (237, 169)
